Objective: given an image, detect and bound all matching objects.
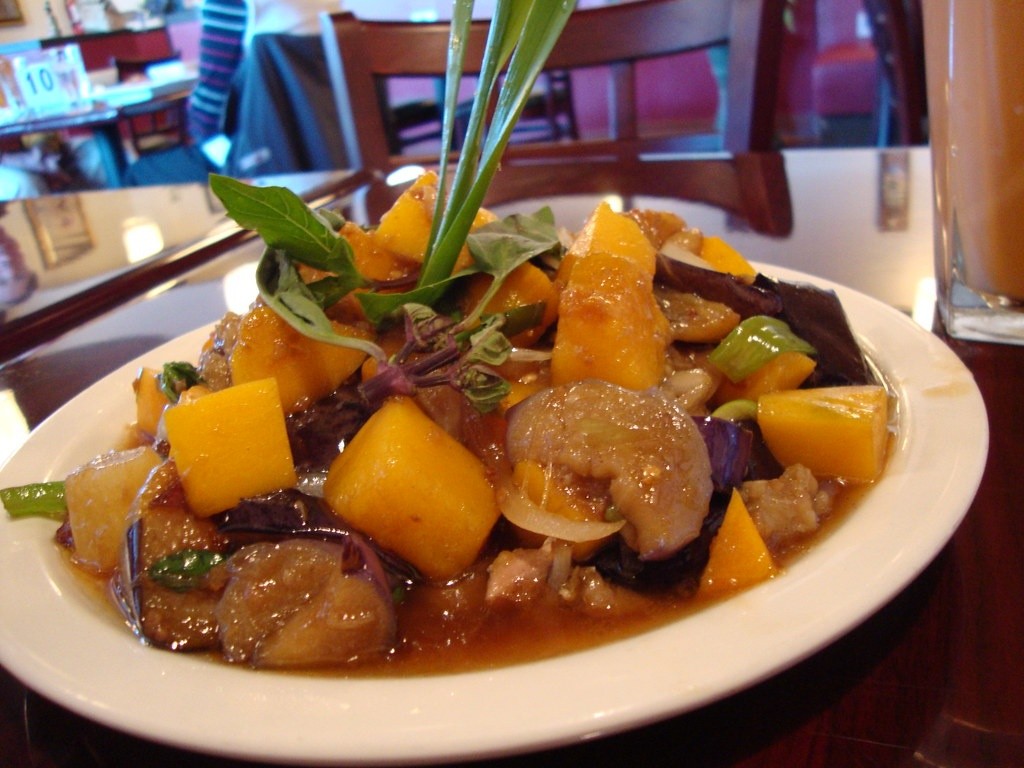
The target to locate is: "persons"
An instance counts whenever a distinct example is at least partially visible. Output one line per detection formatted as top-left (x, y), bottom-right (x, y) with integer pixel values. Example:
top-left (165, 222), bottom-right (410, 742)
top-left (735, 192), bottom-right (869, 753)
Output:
top-left (104, 0), bottom-right (185, 32)
top-left (0, 125), bottom-right (121, 202)
top-left (121, 0), bottom-right (347, 185)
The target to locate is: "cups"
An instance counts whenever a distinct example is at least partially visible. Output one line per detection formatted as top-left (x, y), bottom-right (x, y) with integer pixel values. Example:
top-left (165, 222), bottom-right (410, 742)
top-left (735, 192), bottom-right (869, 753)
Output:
top-left (922, 0), bottom-right (1024, 347)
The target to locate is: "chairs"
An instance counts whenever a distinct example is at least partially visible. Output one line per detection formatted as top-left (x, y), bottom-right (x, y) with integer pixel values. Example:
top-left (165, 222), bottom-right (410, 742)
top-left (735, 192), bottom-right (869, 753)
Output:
top-left (110, 49), bottom-right (189, 160)
top-left (860, 1), bottom-right (924, 148)
top-left (320, 0), bottom-right (784, 173)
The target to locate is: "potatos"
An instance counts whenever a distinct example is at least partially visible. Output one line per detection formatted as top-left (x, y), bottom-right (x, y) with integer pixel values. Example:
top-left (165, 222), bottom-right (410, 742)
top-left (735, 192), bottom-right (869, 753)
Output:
top-left (135, 171), bottom-right (891, 595)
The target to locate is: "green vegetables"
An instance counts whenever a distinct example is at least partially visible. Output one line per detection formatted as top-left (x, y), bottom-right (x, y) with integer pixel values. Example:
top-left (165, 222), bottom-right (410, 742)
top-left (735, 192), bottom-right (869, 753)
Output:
top-left (1, 173), bottom-right (820, 595)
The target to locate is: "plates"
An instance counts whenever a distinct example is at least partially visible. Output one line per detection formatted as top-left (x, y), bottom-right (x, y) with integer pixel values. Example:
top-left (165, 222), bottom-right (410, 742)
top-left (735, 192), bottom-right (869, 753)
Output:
top-left (0, 259), bottom-right (988, 765)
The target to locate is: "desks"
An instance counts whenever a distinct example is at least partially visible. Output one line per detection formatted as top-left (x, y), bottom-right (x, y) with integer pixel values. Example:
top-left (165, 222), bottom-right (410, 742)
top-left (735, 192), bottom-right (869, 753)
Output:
top-left (0, 78), bottom-right (202, 186)
top-left (0, 146), bottom-right (1024, 768)
